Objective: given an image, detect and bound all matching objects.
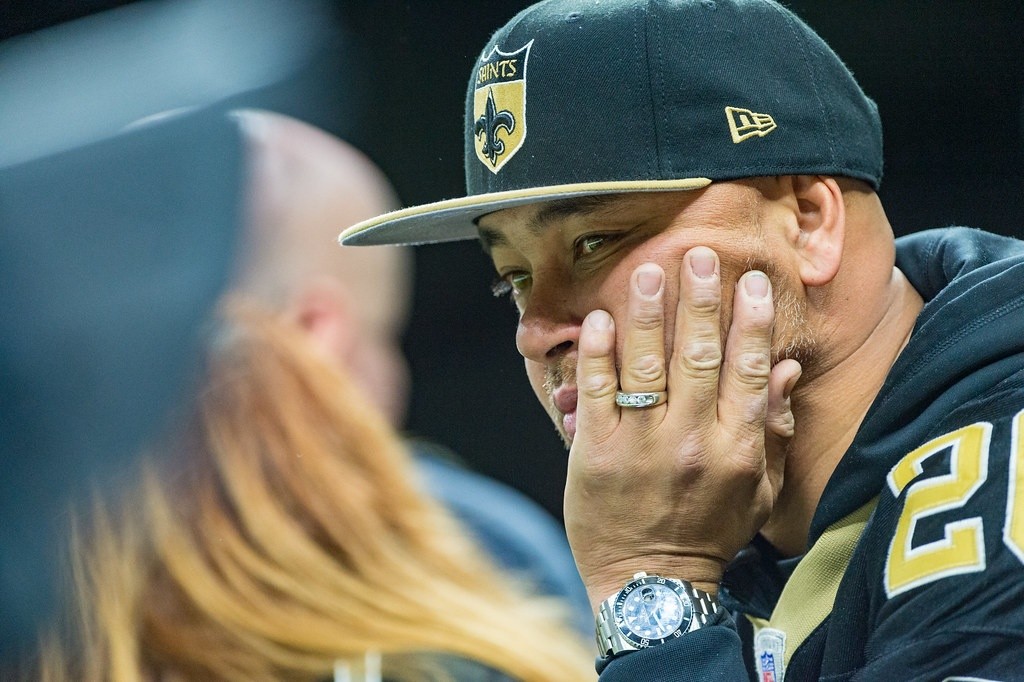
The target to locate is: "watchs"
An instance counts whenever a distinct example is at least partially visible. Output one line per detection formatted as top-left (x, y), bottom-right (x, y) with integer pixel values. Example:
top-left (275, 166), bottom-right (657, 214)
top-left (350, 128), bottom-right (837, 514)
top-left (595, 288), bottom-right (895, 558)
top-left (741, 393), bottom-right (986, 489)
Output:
top-left (594, 571), bottom-right (720, 656)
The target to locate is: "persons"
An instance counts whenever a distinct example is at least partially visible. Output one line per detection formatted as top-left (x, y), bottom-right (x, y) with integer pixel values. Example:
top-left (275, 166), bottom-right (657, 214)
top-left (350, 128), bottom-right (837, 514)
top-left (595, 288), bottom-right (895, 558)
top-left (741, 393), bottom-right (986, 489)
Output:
top-left (0, 1), bottom-right (600, 682)
top-left (336, 0), bottom-right (1023, 682)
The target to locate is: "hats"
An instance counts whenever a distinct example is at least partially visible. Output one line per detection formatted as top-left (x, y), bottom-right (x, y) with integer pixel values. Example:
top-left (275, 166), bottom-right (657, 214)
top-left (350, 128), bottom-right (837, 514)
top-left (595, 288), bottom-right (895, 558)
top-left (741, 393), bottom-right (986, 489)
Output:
top-left (338, 0), bottom-right (883, 247)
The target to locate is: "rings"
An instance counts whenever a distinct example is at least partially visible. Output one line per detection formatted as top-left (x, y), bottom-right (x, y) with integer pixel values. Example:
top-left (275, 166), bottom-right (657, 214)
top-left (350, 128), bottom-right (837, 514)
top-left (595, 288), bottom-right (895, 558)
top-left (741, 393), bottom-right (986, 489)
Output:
top-left (615, 390), bottom-right (666, 408)
